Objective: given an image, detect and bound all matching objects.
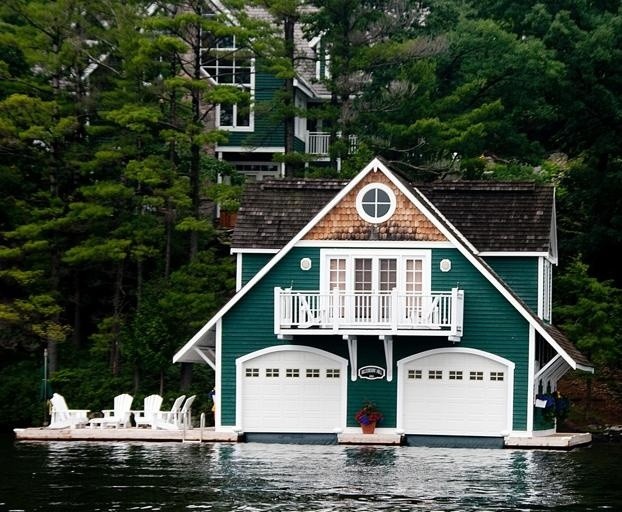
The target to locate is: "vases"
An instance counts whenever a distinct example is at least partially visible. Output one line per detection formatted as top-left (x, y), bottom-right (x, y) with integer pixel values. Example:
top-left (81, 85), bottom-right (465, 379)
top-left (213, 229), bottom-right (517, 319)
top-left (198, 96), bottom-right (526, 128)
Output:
top-left (361, 422), bottom-right (377, 434)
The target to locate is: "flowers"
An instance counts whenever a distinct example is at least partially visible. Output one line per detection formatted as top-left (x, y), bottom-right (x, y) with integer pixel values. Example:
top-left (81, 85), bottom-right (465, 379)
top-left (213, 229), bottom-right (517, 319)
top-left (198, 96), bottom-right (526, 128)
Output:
top-left (354, 400), bottom-right (384, 425)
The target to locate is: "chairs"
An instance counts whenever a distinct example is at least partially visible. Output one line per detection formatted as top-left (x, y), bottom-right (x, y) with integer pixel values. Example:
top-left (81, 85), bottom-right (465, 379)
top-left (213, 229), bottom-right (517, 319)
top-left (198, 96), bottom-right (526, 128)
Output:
top-left (51, 391), bottom-right (197, 431)
